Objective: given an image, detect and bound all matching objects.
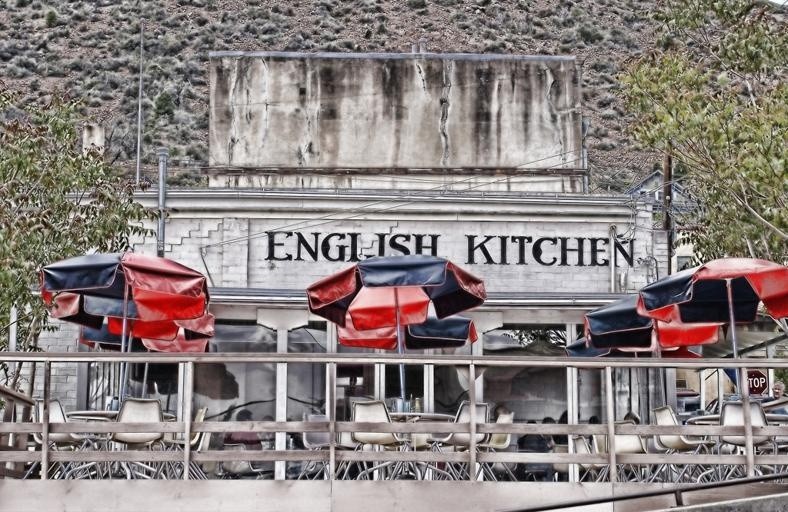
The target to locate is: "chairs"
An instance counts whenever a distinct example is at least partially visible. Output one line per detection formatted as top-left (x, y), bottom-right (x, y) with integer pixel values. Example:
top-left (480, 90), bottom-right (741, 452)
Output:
top-left (338, 417), bottom-right (366, 480)
top-left (420, 398), bottom-right (487, 480)
top-left (464, 412), bottom-right (520, 479)
top-left (720, 403), bottom-right (776, 482)
top-left (651, 405), bottom-right (715, 481)
top-left (298, 412), bottom-right (353, 479)
top-left (603, 421), bottom-right (657, 481)
top-left (489, 420), bottom-right (621, 481)
top-left (26, 395), bottom-right (273, 480)
top-left (351, 401), bottom-right (412, 479)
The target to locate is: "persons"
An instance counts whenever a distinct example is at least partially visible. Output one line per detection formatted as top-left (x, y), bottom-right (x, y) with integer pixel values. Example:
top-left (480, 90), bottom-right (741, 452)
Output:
top-left (487, 404), bottom-right (600, 482)
top-left (224, 411), bottom-right (263, 463)
top-left (761, 381), bottom-right (788, 415)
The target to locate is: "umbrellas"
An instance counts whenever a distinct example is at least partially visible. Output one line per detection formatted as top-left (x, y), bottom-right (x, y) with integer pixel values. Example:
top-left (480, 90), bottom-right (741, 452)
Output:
top-left (566, 337), bottom-right (703, 368)
top-left (636, 257), bottom-right (788, 403)
top-left (306, 253), bottom-right (487, 414)
top-left (583, 290), bottom-right (759, 409)
top-left (49, 293), bottom-right (215, 402)
top-left (79, 318), bottom-right (210, 398)
top-left (41, 252), bottom-right (212, 410)
top-left (332, 302), bottom-right (478, 402)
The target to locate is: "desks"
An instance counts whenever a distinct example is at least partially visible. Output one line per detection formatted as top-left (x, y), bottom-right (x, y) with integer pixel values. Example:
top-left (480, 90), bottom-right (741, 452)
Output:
top-left (682, 411), bottom-right (788, 479)
top-left (370, 410), bottom-right (456, 479)
